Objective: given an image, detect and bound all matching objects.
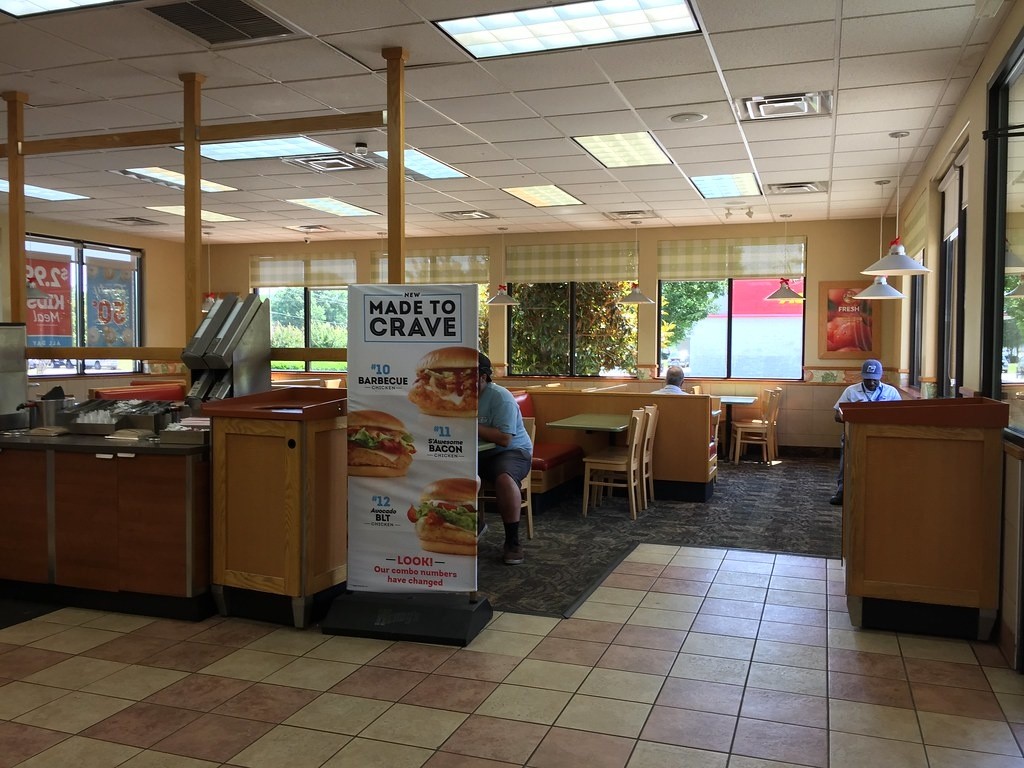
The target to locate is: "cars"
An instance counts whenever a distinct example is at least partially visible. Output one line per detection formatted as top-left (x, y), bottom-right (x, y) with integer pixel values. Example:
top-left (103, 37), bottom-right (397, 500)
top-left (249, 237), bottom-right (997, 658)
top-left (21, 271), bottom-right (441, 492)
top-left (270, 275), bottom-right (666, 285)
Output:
top-left (1001, 355), bottom-right (1008, 373)
top-left (1015, 356), bottom-right (1024, 374)
top-left (63, 359), bottom-right (118, 371)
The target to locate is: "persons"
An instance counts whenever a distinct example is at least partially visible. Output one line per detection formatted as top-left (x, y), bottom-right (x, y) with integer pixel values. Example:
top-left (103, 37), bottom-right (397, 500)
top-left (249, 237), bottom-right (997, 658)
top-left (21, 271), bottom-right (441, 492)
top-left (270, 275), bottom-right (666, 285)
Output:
top-left (651, 366), bottom-right (688, 394)
top-left (830, 359), bottom-right (903, 505)
top-left (476, 352), bottom-right (533, 564)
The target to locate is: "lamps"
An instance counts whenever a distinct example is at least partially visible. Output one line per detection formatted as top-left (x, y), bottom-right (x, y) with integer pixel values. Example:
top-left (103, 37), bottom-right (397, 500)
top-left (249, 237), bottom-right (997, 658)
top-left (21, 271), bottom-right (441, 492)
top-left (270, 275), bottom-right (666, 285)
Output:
top-left (851, 180), bottom-right (908, 300)
top-left (26, 210), bottom-right (52, 299)
top-left (764, 213), bottom-right (806, 299)
top-left (618, 221), bottom-right (656, 304)
top-left (201, 232), bottom-right (216, 312)
top-left (859, 132), bottom-right (932, 276)
top-left (486, 227), bottom-right (521, 305)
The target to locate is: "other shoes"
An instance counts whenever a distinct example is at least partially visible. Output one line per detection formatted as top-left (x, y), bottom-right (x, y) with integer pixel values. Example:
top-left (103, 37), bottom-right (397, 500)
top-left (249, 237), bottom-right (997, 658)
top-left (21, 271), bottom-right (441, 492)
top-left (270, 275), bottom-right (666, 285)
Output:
top-left (830, 490), bottom-right (843, 505)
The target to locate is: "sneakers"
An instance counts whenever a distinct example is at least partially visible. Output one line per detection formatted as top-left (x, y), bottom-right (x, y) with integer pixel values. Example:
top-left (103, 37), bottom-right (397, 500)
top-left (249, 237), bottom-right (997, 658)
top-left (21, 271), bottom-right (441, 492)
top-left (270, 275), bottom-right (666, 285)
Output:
top-left (478, 519), bottom-right (488, 541)
top-left (503, 542), bottom-right (524, 564)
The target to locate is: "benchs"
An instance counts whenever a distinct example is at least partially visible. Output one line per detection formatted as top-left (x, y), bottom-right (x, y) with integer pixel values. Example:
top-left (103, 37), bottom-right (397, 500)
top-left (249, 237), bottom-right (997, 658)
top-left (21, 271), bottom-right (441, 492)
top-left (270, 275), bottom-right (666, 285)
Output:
top-left (511, 389), bottom-right (575, 514)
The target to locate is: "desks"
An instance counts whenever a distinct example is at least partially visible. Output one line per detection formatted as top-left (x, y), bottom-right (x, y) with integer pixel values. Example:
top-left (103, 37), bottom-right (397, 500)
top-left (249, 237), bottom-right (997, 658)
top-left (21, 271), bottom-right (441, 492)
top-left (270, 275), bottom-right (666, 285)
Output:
top-left (712, 395), bottom-right (758, 464)
top-left (546, 414), bottom-right (633, 514)
top-left (478, 441), bottom-right (496, 452)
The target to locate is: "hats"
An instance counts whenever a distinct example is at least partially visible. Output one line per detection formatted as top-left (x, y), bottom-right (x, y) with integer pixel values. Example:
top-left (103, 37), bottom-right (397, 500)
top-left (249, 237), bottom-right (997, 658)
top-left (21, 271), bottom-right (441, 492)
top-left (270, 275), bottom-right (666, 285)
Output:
top-left (861, 359), bottom-right (883, 380)
top-left (478, 353), bottom-right (492, 383)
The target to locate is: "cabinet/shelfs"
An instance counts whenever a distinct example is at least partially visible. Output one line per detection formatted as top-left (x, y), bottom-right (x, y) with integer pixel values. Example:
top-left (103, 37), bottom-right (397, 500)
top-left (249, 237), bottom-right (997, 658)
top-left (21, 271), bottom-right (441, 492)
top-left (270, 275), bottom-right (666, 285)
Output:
top-left (213, 414), bottom-right (348, 629)
top-left (845, 420), bottom-right (1004, 641)
top-left (0, 427), bottom-right (212, 623)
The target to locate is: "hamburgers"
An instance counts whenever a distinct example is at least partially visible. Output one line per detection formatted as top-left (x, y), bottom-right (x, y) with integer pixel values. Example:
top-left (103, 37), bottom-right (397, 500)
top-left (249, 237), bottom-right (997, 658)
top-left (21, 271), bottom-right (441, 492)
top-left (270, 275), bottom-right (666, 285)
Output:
top-left (346, 409), bottom-right (417, 476)
top-left (408, 346), bottom-right (479, 418)
top-left (407, 478), bottom-right (477, 555)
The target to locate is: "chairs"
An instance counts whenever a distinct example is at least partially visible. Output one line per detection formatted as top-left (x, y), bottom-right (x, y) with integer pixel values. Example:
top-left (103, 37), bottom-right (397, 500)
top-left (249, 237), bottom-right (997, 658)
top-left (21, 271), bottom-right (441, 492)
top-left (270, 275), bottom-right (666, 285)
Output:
top-left (690, 386), bottom-right (704, 396)
top-left (582, 408), bottom-right (645, 520)
top-left (519, 417), bottom-right (537, 540)
top-left (599, 404), bottom-right (659, 508)
top-left (729, 392), bottom-right (780, 465)
top-left (739, 386), bottom-right (782, 462)
